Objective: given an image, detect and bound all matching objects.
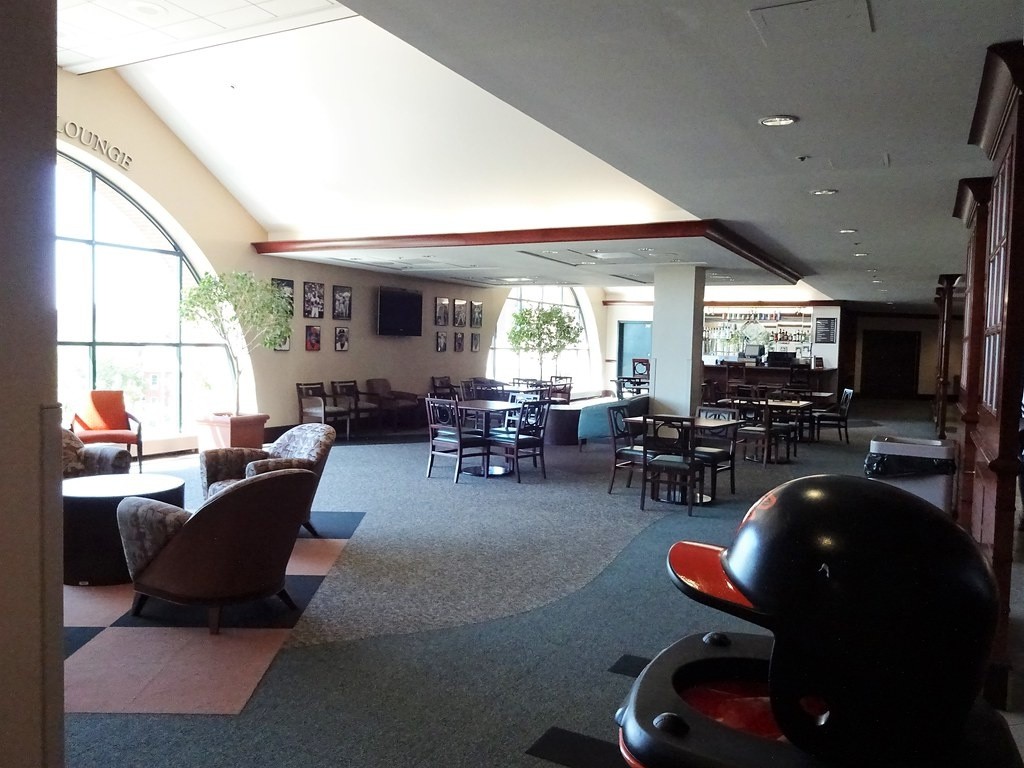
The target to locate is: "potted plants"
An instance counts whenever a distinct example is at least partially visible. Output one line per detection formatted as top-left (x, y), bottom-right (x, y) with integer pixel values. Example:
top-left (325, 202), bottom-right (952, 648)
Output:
top-left (176, 271), bottom-right (297, 450)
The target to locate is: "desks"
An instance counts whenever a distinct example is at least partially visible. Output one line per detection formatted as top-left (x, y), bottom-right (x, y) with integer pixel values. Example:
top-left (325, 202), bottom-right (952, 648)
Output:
top-left (431, 399), bottom-right (535, 478)
top-left (775, 391), bottom-right (834, 401)
top-left (730, 399), bottom-right (815, 457)
top-left (623, 413), bottom-right (745, 502)
top-left (618, 376), bottom-right (649, 378)
top-left (544, 381), bottom-right (572, 387)
top-left (474, 386), bottom-right (547, 393)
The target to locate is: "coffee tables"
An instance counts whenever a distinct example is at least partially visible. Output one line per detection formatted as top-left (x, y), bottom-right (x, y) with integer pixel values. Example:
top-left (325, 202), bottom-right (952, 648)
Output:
top-left (538, 405), bottom-right (584, 445)
top-left (62, 473), bottom-right (186, 586)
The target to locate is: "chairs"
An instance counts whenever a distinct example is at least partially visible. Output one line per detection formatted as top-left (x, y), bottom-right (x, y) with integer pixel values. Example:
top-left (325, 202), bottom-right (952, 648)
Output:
top-left (64, 391), bottom-right (143, 473)
top-left (296, 375), bottom-right (854, 516)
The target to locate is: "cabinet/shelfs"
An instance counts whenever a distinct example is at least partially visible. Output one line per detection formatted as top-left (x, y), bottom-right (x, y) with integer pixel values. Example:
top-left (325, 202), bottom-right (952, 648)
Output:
top-left (705, 313), bottom-right (811, 334)
top-left (934, 39), bottom-right (1023, 717)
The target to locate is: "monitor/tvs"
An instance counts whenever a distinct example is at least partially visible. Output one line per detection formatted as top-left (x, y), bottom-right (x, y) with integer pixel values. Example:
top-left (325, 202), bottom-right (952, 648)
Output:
top-left (376, 285), bottom-right (422, 336)
top-left (745, 345), bottom-right (766, 357)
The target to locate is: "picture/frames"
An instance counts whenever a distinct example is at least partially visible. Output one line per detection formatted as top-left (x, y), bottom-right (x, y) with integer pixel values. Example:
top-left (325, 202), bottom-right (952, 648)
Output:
top-left (435, 299), bottom-right (482, 353)
top-left (270, 278), bottom-right (352, 353)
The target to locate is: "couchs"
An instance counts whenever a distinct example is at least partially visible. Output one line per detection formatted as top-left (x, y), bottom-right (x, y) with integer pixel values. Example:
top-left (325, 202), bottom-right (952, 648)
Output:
top-left (62, 421), bottom-right (336, 635)
top-left (577, 392), bottom-right (650, 450)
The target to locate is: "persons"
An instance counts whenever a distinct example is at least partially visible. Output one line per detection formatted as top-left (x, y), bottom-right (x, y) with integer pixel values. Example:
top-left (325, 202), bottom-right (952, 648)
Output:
top-left (474, 305), bottom-right (481, 325)
top-left (440, 334), bottom-right (445, 350)
top-left (438, 305), bottom-right (448, 324)
top-left (336, 329), bottom-right (348, 350)
top-left (307, 332), bottom-right (319, 350)
top-left (334, 291), bottom-right (346, 316)
top-left (457, 335), bottom-right (462, 350)
top-left (310, 290), bottom-right (319, 318)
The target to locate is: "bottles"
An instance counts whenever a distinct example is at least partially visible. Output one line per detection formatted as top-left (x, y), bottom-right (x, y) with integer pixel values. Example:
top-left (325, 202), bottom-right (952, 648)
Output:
top-left (703, 324), bottom-right (810, 342)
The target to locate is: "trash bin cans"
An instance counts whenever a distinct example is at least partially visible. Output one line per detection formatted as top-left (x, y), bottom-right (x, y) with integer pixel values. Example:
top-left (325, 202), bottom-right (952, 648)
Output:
top-left (868, 433), bottom-right (959, 514)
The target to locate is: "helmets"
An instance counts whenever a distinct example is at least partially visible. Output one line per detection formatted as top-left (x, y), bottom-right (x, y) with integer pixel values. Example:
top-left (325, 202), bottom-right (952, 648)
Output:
top-left (667, 473), bottom-right (1004, 757)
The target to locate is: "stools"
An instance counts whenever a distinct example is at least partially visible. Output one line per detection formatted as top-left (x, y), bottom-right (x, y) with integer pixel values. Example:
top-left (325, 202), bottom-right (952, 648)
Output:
top-left (785, 364), bottom-right (812, 392)
top-left (726, 361), bottom-right (747, 398)
top-left (633, 358), bottom-right (650, 395)
